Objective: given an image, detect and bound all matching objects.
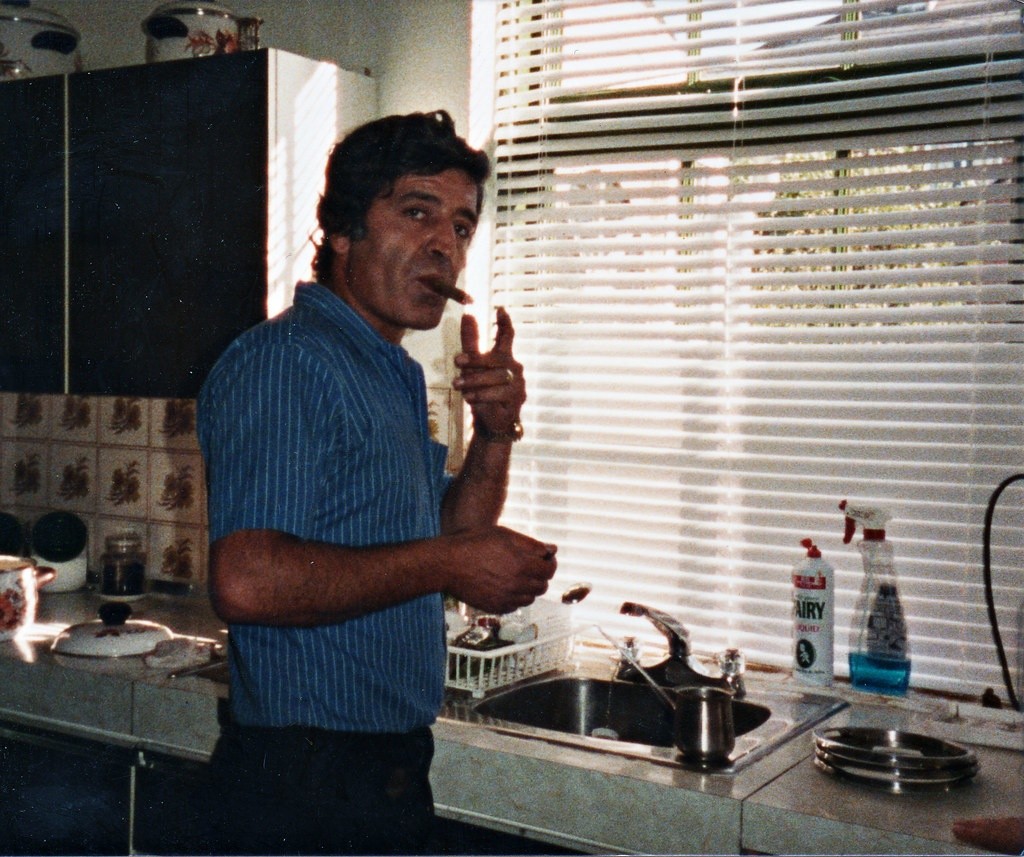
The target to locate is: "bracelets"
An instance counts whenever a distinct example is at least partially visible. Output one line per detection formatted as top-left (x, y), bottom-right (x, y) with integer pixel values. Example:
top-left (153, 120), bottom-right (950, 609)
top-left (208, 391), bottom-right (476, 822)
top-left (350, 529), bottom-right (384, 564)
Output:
top-left (470, 415), bottom-right (524, 441)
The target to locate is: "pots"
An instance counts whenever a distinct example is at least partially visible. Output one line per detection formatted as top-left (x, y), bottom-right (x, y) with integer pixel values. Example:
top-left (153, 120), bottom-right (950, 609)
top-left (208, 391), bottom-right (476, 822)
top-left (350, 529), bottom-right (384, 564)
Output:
top-left (0, 0), bottom-right (81, 81)
top-left (141, 0), bottom-right (243, 63)
top-left (0, 555), bottom-right (56, 643)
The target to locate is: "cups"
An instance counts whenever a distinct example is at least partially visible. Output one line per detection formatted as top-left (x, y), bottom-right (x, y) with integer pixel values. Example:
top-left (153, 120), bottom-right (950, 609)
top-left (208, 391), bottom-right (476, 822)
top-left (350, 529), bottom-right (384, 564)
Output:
top-left (676, 687), bottom-right (735, 769)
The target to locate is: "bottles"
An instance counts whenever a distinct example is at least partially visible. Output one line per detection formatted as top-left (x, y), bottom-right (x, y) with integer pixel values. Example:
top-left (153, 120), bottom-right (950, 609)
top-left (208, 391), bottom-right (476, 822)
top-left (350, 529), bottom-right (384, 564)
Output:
top-left (101, 534), bottom-right (146, 596)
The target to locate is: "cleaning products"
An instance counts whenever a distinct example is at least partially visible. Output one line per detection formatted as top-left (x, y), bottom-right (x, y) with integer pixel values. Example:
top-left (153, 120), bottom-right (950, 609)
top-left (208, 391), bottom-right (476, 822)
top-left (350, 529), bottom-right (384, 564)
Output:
top-left (786, 538), bottom-right (841, 690)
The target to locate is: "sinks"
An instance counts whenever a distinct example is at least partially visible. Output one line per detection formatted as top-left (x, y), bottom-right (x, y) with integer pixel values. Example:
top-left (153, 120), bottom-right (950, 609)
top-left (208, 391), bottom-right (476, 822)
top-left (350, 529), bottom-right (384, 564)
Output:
top-left (470, 674), bottom-right (773, 749)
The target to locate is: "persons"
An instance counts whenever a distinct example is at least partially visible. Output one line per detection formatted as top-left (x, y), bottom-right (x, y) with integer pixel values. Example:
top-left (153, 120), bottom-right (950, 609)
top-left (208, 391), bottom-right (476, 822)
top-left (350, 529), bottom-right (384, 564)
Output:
top-left (193, 105), bottom-right (558, 856)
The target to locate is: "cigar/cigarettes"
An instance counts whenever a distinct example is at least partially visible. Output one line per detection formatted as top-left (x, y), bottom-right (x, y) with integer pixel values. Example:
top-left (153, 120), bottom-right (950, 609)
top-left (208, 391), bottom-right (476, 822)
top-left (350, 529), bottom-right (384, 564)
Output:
top-left (421, 277), bottom-right (475, 305)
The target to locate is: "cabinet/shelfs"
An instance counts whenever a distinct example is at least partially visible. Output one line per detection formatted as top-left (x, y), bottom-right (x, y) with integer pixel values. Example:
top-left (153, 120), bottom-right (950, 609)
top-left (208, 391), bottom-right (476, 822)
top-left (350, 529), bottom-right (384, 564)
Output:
top-left (0, 737), bottom-right (130, 856)
top-left (0, 47), bottom-right (269, 399)
top-left (132, 766), bottom-right (212, 855)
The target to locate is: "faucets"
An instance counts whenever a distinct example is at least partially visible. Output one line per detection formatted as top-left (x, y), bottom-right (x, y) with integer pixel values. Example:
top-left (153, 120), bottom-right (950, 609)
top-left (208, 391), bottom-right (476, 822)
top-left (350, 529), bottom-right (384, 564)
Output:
top-left (619, 599), bottom-right (693, 687)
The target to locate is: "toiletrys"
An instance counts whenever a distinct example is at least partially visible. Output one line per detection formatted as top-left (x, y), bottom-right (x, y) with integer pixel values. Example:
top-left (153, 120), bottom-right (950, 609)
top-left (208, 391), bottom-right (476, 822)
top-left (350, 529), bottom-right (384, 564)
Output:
top-left (839, 498), bottom-right (912, 696)
